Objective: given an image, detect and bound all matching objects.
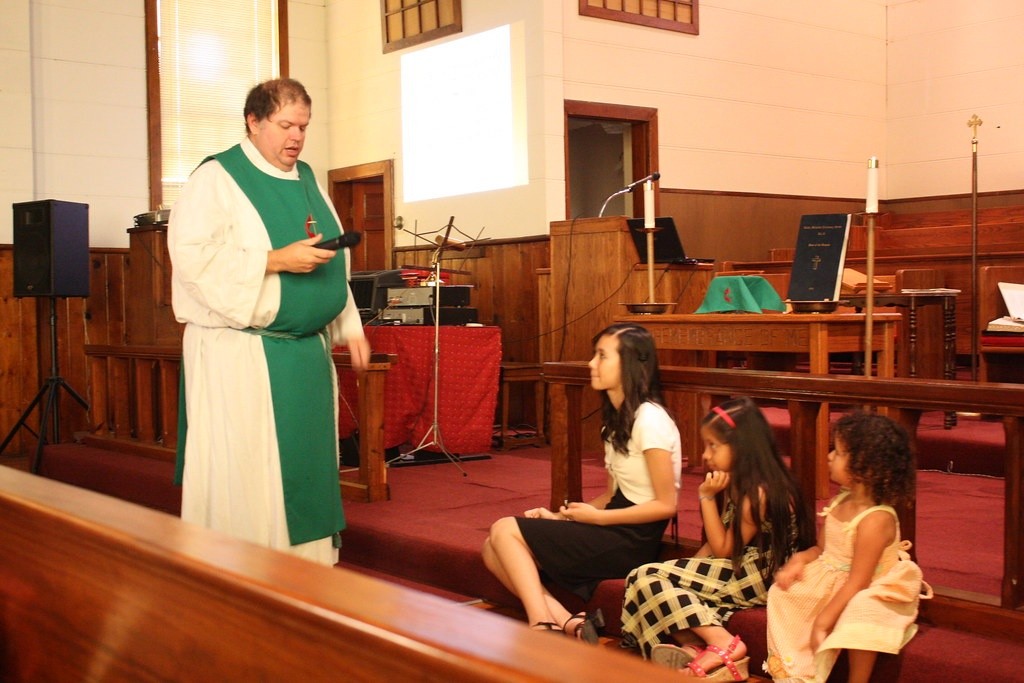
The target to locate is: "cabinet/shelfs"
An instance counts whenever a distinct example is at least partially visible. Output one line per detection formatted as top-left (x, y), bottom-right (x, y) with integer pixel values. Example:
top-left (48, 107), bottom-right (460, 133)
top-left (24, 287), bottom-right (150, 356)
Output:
top-left (127, 225), bottom-right (186, 348)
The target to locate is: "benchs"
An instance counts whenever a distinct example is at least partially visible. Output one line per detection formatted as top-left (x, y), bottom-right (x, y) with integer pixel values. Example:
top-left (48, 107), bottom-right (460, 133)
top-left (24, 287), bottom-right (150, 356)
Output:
top-left (978, 334), bottom-right (1024, 416)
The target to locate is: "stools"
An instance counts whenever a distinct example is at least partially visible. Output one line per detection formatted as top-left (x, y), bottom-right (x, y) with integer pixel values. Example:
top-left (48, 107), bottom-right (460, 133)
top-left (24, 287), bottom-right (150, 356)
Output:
top-left (493, 362), bottom-right (544, 452)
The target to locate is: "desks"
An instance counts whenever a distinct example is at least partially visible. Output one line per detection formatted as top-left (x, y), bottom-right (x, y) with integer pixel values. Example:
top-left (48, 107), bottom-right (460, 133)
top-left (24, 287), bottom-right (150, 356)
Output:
top-left (839, 290), bottom-right (960, 430)
top-left (613, 314), bottom-right (903, 564)
top-left (332, 326), bottom-right (502, 468)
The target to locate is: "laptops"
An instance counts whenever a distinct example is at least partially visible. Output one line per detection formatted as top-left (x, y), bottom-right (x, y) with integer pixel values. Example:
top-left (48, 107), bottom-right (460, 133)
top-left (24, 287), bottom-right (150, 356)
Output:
top-left (626, 216), bottom-right (714, 264)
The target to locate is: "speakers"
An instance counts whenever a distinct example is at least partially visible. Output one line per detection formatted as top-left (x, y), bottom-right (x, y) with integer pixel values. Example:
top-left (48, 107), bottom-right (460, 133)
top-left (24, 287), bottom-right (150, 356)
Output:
top-left (13, 199), bottom-right (90, 298)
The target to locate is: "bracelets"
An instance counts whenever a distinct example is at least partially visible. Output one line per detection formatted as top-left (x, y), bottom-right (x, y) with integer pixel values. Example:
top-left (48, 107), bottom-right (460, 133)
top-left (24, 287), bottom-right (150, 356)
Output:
top-left (698, 496), bottom-right (716, 500)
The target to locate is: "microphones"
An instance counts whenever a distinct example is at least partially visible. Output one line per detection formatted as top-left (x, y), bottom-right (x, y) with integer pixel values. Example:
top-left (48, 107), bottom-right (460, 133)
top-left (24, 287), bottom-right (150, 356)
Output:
top-left (311, 232), bottom-right (361, 250)
top-left (627, 172), bottom-right (660, 188)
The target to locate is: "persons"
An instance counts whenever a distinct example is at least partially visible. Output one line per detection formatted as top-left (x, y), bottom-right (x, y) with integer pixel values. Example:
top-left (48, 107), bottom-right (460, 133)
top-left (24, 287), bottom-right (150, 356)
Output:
top-left (761, 413), bottom-right (934, 683)
top-left (619, 397), bottom-right (807, 683)
top-left (480, 320), bottom-right (682, 646)
top-left (166, 77), bottom-right (371, 567)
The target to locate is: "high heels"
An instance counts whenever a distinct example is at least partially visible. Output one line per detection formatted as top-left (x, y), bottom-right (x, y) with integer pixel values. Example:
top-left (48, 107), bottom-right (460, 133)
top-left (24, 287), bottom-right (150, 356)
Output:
top-left (531, 622), bottom-right (563, 632)
top-left (651, 644), bottom-right (703, 669)
top-left (563, 608), bottom-right (605, 646)
top-left (678, 635), bottom-right (750, 682)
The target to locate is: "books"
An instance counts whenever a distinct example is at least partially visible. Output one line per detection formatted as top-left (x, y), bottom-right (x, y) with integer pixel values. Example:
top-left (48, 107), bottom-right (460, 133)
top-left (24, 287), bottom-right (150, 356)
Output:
top-left (983, 281), bottom-right (1024, 332)
top-left (785, 213), bottom-right (853, 302)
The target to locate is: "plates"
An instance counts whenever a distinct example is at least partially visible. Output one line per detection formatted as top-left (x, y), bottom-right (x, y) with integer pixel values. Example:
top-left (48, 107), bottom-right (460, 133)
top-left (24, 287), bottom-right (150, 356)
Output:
top-left (618, 301), bottom-right (679, 316)
top-left (783, 299), bottom-right (847, 314)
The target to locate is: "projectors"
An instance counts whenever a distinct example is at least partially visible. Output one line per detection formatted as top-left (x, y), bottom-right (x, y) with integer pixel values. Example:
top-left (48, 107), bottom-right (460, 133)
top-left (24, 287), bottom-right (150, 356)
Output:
top-left (136, 209), bottom-right (171, 227)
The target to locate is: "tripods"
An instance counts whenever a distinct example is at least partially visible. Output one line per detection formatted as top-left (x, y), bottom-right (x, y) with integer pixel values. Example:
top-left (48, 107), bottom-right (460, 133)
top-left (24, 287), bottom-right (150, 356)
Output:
top-left (0, 298), bottom-right (90, 475)
top-left (385, 215), bottom-right (486, 476)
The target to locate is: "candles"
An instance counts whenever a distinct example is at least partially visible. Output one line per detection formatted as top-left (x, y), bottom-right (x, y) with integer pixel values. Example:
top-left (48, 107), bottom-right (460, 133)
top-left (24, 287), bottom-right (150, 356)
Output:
top-left (865, 155), bottom-right (878, 213)
top-left (643, 179), bottom-right (655, 229)
